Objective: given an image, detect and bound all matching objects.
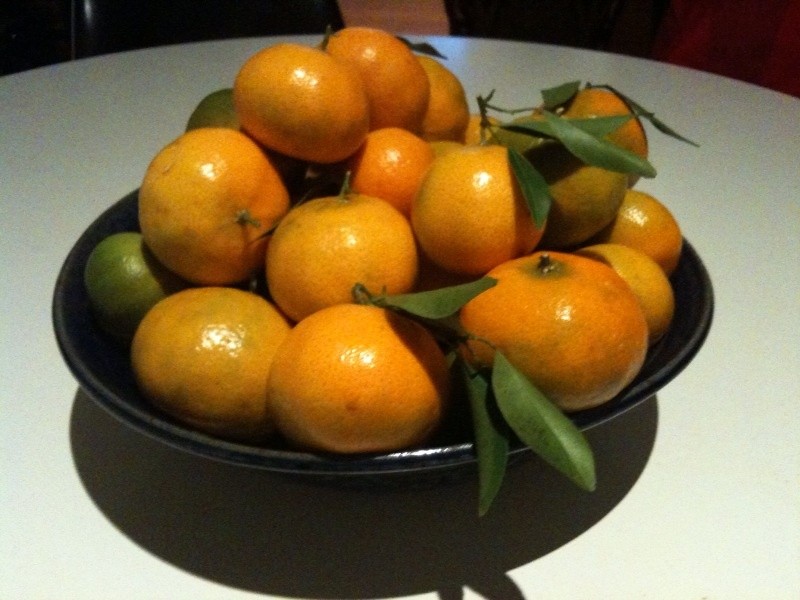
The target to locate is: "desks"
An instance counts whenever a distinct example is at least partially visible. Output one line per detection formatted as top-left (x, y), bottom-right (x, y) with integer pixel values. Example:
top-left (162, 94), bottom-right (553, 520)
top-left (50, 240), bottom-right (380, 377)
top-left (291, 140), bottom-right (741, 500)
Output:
top-left (2, 34), bottom-right (800, 600)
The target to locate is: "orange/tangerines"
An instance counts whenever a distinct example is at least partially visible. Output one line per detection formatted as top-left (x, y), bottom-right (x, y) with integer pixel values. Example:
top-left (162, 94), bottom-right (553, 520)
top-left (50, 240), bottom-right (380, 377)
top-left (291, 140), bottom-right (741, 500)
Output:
top-left (82, 26), bottom-right (684, 456)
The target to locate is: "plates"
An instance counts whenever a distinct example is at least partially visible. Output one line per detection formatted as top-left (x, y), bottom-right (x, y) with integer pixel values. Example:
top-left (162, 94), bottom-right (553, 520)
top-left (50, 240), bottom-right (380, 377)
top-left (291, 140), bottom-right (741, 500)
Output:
top-left (52, 187), bottom-right (715, 472)
